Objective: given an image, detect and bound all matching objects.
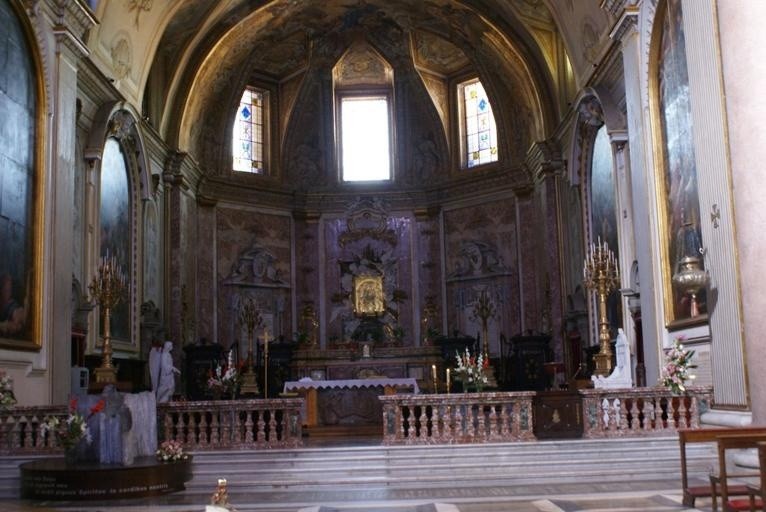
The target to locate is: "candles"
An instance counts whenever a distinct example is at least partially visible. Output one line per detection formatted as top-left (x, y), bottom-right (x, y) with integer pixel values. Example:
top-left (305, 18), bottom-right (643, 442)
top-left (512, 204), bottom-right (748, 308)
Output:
top-left (432, 364), bottom-right (450, 383)
top-left (583, 234), bottom-right (619, 278)
top-left (91, 247), bottom-right (126, 289)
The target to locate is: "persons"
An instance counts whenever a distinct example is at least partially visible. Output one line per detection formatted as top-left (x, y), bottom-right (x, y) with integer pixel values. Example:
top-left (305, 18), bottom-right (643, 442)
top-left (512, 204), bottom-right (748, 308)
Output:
top-left (615, 327), bottom-right (631, 371)
top-left (155, 340), bottom-right (182, 402)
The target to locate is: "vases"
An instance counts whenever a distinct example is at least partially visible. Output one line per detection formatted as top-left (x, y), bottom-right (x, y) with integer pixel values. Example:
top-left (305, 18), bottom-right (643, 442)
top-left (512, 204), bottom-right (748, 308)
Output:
top-left (64, 442), bottom-right (97, 470)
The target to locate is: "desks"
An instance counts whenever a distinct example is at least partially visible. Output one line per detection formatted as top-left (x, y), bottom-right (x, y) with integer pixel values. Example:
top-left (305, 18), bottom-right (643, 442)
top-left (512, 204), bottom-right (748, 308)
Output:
top-left (283, 378), bottom-right (420, 428)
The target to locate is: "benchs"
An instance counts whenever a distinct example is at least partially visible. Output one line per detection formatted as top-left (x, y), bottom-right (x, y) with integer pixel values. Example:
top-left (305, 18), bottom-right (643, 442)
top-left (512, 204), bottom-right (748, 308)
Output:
top-left (677, 427), bottom-right (766, 512)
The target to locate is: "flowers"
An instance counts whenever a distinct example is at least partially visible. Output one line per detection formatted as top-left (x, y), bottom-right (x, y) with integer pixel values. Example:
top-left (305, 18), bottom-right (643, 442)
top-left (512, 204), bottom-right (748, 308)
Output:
top-left (657, 334), bottom-right (697, 394)
top-left (454, 347), bottom-right (488, 393)
top-left (0, 368), bottom-right (18, 411)
top-left (206, 349), bottom-right (239, 392)
top-left (37, 394), bottom-right (107, 446)
top-left (155, 438), bottom-right (189, 462)
